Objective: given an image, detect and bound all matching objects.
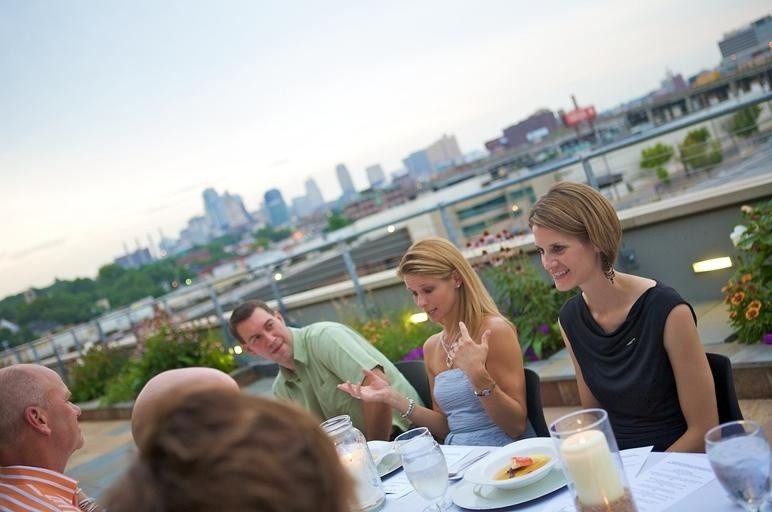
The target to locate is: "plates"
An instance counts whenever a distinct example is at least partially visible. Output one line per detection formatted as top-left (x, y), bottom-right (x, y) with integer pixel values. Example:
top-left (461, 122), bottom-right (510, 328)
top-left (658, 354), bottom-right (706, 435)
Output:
top-left (452, 481), bottom-right (567, 509)
top-left (368, 440), bottom-right (407, 481)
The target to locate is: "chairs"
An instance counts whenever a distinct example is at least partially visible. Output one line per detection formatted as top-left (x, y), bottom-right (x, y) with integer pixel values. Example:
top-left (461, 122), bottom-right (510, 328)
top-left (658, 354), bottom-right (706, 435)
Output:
top-left (394, 361), bottom-right (444, 445)
top-left (524, 368), bottom-right (551, 437)
top-left (705, 352), bottom-right (746, 437)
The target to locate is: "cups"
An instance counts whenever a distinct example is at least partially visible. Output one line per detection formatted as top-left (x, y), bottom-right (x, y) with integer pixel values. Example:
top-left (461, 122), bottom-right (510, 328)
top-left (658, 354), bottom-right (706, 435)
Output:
top-left (551, 410), bottom-right (645, 510)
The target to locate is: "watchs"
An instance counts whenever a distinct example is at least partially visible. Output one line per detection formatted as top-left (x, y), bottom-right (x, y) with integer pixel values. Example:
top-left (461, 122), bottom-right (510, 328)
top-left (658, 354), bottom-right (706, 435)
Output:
top-left (475, 382), bottom-right (497, 398)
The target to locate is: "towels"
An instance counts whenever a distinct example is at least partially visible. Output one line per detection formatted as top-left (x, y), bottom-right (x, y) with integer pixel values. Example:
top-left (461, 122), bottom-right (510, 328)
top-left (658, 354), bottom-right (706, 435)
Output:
top-left (366, 440), bottom-right (401, 477)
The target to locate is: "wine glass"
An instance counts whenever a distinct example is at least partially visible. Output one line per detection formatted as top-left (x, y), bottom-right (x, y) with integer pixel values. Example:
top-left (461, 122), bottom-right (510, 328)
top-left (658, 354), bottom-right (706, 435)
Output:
top-left (394, 426), bottom-right (449, 511)
top-left (704, 421), bottom-right (771, 511)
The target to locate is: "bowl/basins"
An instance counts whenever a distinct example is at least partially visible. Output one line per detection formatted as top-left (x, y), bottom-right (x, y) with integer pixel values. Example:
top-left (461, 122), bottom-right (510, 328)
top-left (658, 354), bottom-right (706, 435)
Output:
top-left (468, 438), bottom-right (561, 489)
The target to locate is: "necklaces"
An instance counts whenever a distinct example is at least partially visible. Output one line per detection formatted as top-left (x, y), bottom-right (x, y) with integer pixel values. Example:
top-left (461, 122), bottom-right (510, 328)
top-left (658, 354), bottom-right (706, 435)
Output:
top-left (440, 329), bottom-right (461, 369)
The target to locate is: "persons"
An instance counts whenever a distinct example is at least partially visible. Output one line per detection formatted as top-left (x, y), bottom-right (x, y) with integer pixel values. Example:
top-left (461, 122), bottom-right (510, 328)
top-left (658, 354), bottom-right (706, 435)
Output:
top-left (100, 389), bottom-right (363, 512)
top-left (529, 182), bottom-right (721, 455)
top-left (0, 363), bottom-right (109, 512)
top-left (336, 236), bottom-right (537, 448)
top-left (229, 300), bottom-right (426, 443)
top-left (131, 367), bottom-right (239, 452)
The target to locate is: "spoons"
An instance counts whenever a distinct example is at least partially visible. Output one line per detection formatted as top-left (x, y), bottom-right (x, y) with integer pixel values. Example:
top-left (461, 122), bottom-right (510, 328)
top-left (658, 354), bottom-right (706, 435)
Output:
top-left (448, 450), bottom-right (490, 481)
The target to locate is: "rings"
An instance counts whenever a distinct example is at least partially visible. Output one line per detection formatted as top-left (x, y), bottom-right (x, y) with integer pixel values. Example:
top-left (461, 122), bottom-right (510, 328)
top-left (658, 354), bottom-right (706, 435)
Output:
top-left (451, 344), bottom-right (458, 348)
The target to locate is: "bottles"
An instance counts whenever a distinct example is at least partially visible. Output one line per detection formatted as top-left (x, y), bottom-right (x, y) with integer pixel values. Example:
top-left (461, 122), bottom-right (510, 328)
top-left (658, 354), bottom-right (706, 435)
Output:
top-left (317, 414), bottom-right (387, 512)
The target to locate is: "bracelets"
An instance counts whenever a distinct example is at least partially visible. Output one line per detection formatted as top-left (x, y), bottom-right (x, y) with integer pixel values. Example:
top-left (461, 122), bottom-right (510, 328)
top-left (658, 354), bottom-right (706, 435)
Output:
top-left (78, 498), bottom-right (93, 511)
top-left (86, 503), bottom-right (98, 512)
top-left (400, 398), bottom-right (415, 419)
